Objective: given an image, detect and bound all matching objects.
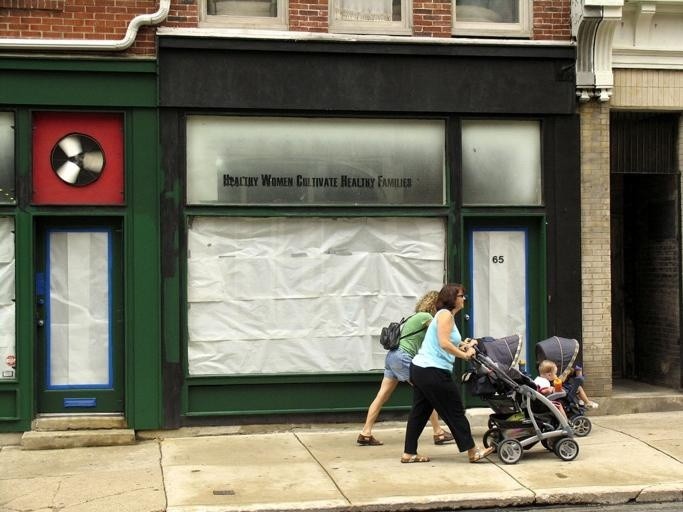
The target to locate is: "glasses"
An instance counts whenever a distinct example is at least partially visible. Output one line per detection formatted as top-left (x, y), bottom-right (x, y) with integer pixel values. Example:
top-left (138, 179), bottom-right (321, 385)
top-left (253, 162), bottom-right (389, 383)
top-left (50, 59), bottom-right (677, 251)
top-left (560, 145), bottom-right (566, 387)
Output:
top-left (457, 294), bottom-right (464, 297)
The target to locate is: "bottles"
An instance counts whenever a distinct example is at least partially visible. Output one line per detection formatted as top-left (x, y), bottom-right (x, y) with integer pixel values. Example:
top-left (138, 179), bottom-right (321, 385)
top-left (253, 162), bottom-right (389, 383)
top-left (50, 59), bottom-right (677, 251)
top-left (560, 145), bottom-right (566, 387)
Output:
top-left (553, 378), bottom-right (562, 392)
top-left (518, 360), bottom-right (525, 373)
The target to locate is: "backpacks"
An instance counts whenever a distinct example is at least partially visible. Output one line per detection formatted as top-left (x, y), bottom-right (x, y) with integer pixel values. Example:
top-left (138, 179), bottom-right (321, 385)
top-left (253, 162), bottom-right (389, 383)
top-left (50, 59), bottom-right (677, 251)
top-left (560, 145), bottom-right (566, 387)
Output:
top-left (379, 323), bottom-right (400, 351)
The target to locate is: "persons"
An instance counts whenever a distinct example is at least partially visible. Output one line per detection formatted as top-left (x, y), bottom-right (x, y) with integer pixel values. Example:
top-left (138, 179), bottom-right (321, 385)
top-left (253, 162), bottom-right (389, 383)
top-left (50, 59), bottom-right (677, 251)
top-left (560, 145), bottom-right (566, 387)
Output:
top-left (356, 289), bottom-right (480, 446)
top-left (399, 282), bottom-right (497, 462)
top-left (564, 366), bottom-right (600, 411)
top-left (530, 359), bottom-right (574, 427)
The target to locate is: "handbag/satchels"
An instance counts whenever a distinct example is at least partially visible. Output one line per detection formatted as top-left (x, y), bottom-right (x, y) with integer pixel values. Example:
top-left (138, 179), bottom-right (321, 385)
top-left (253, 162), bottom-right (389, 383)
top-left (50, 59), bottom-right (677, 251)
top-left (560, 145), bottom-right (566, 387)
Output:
top-left (471, 375), bottom-right (496, 395)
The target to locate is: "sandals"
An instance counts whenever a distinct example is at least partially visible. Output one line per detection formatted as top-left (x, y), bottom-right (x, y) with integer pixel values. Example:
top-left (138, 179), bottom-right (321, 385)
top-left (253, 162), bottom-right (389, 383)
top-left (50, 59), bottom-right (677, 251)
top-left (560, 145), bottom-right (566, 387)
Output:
top-left (584, 401), bottom-right (599, 410)
top-left (578, 400), bottom-right (585, 406)
top-left (401, 454), bottom-right (430, 463)
top-left (356, 433), bottom-right (384, 446)
top-left (468, 446), bottom-right (495, 463)
top-left (433, 429), bottom-right (455, 445)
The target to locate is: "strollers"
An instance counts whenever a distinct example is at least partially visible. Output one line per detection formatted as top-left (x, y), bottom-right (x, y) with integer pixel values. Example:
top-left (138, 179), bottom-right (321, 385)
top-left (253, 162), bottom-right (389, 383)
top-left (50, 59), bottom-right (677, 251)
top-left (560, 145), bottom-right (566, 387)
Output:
top-left (461, 334), bottom-right (591, 463)
